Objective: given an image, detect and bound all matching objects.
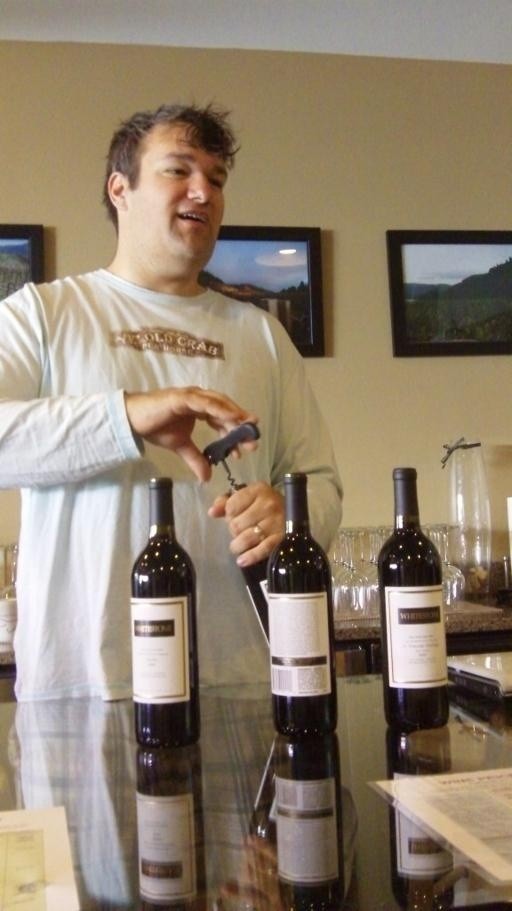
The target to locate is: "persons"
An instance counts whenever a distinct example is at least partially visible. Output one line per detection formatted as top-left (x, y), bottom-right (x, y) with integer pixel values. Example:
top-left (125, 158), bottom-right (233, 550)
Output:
top-left (0, 97), bottom-right (345, 904)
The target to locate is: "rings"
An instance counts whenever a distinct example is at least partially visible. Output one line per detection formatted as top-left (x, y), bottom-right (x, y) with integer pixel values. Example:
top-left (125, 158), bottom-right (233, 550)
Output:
top-left (252, 524), bottom-right (269, 546)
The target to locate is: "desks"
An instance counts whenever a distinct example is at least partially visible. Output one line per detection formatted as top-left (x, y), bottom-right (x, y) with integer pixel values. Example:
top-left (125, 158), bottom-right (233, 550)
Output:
top-left (332, 600), bottom-right (512, 642)
top-left (0, 673), bottom-right (511, 910)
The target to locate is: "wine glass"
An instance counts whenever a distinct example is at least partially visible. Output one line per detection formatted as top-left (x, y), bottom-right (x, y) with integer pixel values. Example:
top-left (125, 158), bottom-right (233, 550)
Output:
top-left (422, 523), bottom-right (466, 614)
top-left (329, 526), bottom-right (395, 619)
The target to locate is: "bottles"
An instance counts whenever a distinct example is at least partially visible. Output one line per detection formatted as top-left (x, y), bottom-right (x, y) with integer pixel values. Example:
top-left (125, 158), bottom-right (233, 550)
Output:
top-left (129, 473), bottom-right (197, 746)
top-left (268, 470), bottom-right (337, 737)
top-left (247, 736), bottom-right (276, 859)
top-left (373, 469), bottom-right (447, 727)
top-left (274, 739), bottom-right (344, 907)
top-left (385, 728), bottom-right (454, 907)
top-left (133, 751), bottom-right (205, 909)
top-left (226, 482), bottom-right (268, 639)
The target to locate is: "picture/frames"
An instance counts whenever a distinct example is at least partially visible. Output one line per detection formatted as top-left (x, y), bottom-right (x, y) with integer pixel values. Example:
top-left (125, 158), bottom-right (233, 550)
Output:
top-left (385, 229), bottom-right (512, 358)
top-left (195, 225), bottom-right (326, 358)
top-left (0, 223), bottom-right (46, 302)
